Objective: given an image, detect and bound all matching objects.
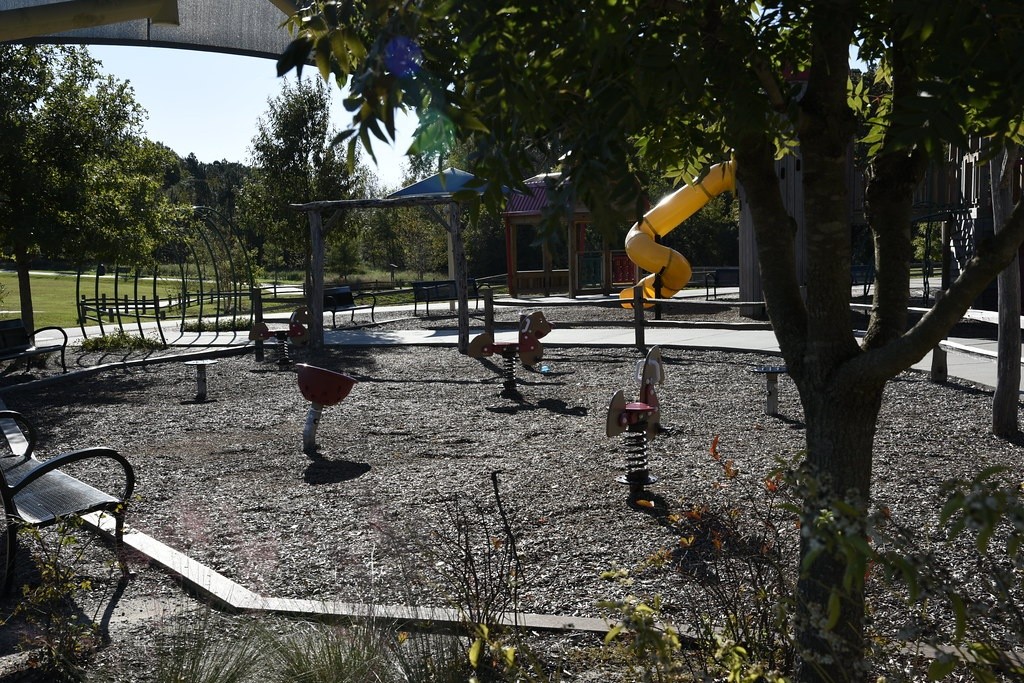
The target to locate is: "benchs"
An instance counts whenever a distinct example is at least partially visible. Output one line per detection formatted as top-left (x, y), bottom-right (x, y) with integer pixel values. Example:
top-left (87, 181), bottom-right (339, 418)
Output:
top-left (411, 279), bottom-right (490, 317)
top-left (0, 318), bottom-right (68, 372)
top-left (850, 265), bottom-right (875, 295)
top-left (0, 410), bottom-right (134, 601)
top-left (684, 271), bottom-right (718, 301)
top-left (322, 284), bottom-right (376, 328)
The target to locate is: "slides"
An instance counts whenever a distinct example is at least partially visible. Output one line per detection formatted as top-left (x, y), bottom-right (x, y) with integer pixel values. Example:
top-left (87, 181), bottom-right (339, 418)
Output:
top-left (619, 160), bottom-right (733, 309)
